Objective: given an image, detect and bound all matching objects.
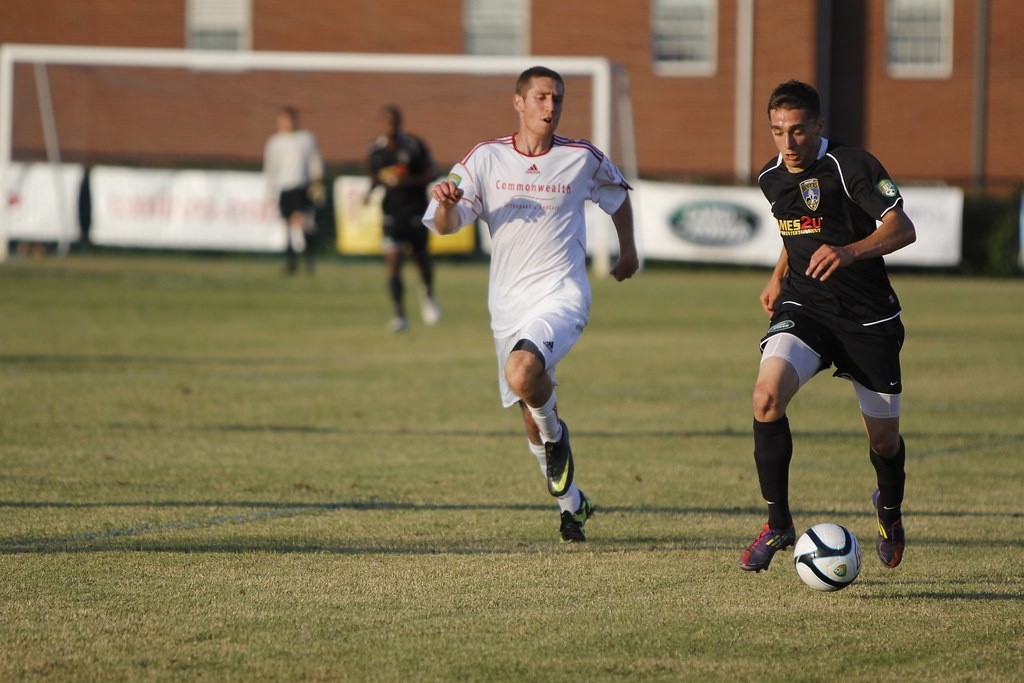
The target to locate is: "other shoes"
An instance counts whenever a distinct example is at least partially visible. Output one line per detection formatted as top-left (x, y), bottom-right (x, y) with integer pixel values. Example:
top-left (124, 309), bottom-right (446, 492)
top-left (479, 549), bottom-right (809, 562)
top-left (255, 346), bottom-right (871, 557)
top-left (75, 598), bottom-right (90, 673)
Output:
top-left (423, 298), bottom-right (440, 324)
top-left (391, 314), bottom-right (407, 337)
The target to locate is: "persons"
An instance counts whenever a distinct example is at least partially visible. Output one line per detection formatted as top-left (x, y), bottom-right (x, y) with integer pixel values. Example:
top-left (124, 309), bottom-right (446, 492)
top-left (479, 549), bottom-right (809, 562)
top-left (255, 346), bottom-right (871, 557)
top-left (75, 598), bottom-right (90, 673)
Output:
top-left (259, 106), bottom-right (327, 275)
top-left (734, 80), bottom-right (917, 574)
top-left (362, 103), bottom-right (443, 333)
top-left (423, 65), bottom-right (638, 543)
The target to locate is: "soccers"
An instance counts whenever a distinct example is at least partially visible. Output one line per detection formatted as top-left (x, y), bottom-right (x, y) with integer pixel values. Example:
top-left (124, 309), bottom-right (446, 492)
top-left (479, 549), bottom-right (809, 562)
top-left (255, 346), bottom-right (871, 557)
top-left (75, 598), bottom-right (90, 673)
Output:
top-left (790, 522), bottom-right (864, 592)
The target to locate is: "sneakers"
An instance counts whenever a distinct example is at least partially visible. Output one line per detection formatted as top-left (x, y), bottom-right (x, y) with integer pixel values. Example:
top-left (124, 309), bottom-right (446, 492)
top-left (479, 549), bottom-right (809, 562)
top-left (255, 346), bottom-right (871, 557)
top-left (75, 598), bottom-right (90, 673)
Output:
top-left (544, 418), bottom-right (574, 495)
top-left (560, 488), bottom-right (592, 542)
top-left (739, 522), bottom-right (797, 574)
top-left (872, 488), bottom-right (904, 569)
